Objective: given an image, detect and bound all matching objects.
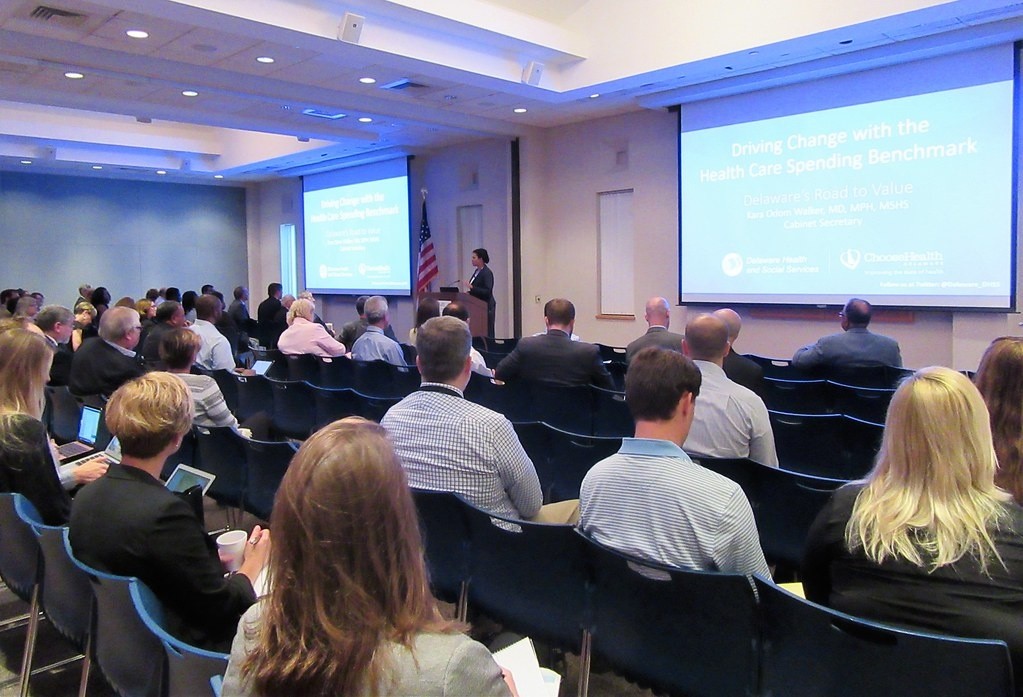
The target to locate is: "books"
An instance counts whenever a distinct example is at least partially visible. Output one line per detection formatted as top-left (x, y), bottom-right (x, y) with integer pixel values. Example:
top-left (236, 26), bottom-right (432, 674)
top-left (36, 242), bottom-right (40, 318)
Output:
top-left (491, 636), bottom-right (546, 697)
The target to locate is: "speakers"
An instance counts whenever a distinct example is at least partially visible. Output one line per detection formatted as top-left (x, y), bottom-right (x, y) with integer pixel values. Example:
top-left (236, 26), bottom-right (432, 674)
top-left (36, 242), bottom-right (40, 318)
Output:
top-left (337, 12), bottom-right (364, 45)
top-left (522, 61), bottom-right (544, 87)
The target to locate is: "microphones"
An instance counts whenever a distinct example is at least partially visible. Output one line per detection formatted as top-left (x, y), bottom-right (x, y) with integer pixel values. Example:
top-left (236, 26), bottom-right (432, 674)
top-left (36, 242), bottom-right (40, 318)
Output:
top-left (448, 279), bottom-right (461, 286)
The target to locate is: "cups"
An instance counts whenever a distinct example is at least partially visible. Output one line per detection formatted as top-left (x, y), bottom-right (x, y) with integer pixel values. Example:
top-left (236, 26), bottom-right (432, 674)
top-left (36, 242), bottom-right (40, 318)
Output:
top-left (216, 529), bottom-right (247, 576)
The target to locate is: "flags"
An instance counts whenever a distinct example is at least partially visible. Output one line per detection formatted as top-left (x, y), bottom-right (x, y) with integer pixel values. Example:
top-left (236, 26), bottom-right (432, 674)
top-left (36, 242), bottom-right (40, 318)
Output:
top-left (417, 198), bottom-right (438, 292)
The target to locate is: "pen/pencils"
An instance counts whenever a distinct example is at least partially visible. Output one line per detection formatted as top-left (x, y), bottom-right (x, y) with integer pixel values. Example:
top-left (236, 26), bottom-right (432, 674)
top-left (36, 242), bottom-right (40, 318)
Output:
top-left (250, 534), bottom-right (262, 544)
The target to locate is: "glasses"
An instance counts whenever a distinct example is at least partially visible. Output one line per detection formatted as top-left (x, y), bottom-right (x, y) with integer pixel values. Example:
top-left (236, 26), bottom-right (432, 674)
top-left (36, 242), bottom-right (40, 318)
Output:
top-left (838, 312), bottom-right (844, 318)
top-left (134, 324), bottom-right (144, 334)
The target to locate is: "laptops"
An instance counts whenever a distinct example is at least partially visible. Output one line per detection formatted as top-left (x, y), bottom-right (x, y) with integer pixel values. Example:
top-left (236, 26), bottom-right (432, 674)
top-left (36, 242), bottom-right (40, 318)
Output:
top-left (164, 463), bottom-right (216, 495)
top-left (440, 287), bottom-right (459, 293)
top-left (61, 436), bottom-right (122, 474)
top-left (250, 359), bottom-right (275, 376)
top-left (57, 404), bottom-right (104, 460)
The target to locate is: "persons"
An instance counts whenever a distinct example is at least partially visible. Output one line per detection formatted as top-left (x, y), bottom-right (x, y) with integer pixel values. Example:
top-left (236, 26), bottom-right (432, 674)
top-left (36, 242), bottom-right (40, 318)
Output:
top-left (792, 298), bottom-right (902, 370)
top-left (463, 248), bottom-right (497, 340)
top-left (0, 328), bottom-right (110, 527)
top-left (496, 297), bottom-right (615, 390)
top-left (796, 366), bottom-right (1023, 697)
top-left (221, 417), bottom-right (519, 697)
top-left (68, 371), bottom-right (272, 655)
top-left (711, 308), bottom-right (764, 398)
top-left (626, 296), bottom-right (685, 359)
top-left (579, 345), bottom-right (774, 584)
top-left (969, 336), bottom-right (1023, 507)
top-left (442, 302), bottom-right (495, 379)
top-left (379, 315), bottom-right (581, 532)
top-left (0, 282), bottom-right (439, 438)
top-left (677, 313), bottom-right (782, 470)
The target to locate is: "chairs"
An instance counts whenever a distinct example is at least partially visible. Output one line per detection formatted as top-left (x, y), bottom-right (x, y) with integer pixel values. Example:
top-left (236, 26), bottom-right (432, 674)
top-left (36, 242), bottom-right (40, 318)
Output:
top-left (1, 335), bottom-right (1016, 697)
